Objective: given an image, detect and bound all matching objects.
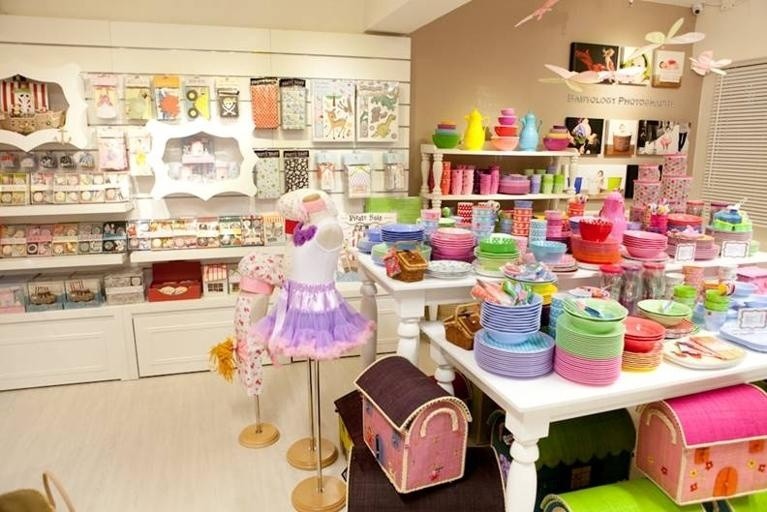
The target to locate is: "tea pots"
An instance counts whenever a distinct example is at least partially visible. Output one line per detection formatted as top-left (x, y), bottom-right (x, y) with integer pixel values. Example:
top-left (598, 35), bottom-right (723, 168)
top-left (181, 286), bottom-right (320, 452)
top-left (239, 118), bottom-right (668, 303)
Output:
top-left (459, 107), bottom-right (489, 151)
top-left (518, 112), bottom-right (542, 151)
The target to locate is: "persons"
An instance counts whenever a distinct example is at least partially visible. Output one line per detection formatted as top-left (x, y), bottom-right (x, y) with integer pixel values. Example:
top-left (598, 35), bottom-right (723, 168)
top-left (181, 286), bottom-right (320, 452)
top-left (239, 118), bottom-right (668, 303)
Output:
top-left (572, 118), bottom-right (600, 155)
top-left (587, 170), bottom-right (603, 195)
top-left (249, 193), bottom-right (376, 362)
top-left (230, 252), bottom-right (285, 396)
top-left (638, 121), bottom-right (690, 154)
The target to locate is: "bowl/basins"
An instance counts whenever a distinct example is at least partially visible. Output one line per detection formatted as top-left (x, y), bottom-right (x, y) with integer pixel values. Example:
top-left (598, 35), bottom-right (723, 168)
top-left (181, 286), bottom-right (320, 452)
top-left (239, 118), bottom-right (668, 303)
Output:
top-left (542, 123), bottom-right (571, 151)
top-left (431, 120), bottom-right (460, 148)
top-left (479, 265), bottom-right (767, 352)
top-left (622, 230), bottom-right (667, 257)
top-left (415, 201), bottom-right (613, 242)
top-left (491, 109), bottom-right (519, 151)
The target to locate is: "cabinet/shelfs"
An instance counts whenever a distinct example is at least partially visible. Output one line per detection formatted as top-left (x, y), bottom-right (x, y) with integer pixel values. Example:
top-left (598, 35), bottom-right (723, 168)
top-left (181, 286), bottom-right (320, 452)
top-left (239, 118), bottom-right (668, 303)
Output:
top-left (416, 143), bottom-right (581, 226)
top-left (2, 64), bottom-right (414, 391)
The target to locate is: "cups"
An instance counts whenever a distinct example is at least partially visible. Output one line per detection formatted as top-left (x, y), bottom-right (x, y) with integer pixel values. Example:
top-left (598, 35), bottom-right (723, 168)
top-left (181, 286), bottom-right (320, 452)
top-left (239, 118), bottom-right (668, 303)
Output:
top-left (525, 167), bottom-right (564, 194)
top-left (587, 176), bottom-right (603, 194)
top-left (606, 176), bottom-right (622, 192)
top-left (567, 177), bottom-right (582, 195)
top-left (612, 134), bottom-right (630, 152)
top-left (428, 162), bottom-right (500, 195)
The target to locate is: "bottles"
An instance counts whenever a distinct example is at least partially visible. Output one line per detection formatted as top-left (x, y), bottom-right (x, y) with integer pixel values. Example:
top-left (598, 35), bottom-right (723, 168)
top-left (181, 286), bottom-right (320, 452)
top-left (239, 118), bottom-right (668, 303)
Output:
top-left (686, 201), bottom-right (729, 232)
top-left (599, 264), bottom-right (666, 307)
top-left (600, 192), bottom-right (627, 241)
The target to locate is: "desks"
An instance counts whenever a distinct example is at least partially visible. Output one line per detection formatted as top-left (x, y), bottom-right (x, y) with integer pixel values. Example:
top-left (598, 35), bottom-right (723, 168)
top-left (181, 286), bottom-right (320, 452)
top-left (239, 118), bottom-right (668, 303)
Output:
top-left (348, 248), bottom-right (766, 511)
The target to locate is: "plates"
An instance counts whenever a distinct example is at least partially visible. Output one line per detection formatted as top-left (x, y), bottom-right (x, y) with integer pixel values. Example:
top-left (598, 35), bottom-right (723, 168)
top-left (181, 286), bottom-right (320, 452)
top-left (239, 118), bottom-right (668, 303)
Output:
top-left (473, 313), bottom-right (665, 387)
top-left (661, 320), bottom-right (767, 370)
top-left (497, 176), bottom-right (530, 194)
top-left (667, 212), bottom-right (759, 260)
top-left (354, 222), bottom-right (622, 284)
